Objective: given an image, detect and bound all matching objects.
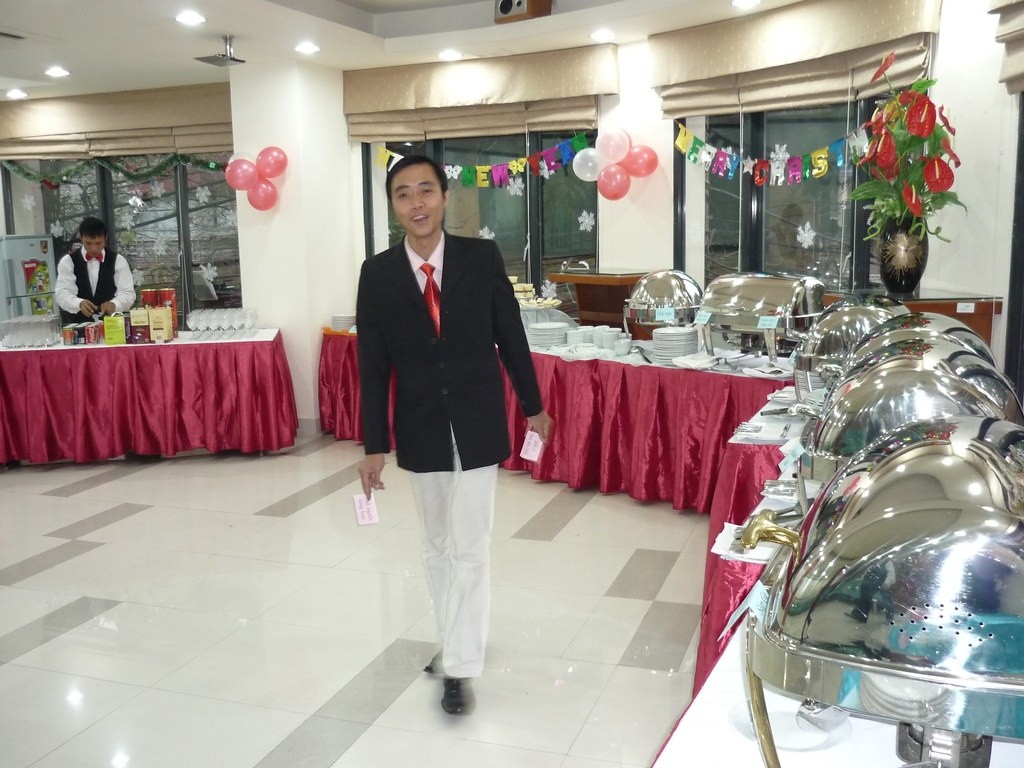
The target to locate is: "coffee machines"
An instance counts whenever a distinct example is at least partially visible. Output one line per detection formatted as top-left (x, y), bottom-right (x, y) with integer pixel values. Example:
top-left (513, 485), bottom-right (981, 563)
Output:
top-left (141, 288), bottom-right (178, 338)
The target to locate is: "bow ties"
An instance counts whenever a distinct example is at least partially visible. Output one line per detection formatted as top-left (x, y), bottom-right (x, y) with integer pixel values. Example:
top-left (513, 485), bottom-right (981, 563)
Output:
top-left (86, 252), bottom-right (102, 262)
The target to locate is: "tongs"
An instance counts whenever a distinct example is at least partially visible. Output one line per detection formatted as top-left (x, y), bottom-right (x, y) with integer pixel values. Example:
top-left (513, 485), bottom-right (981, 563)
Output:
top-left (780, 423), bottom-right (791, 438)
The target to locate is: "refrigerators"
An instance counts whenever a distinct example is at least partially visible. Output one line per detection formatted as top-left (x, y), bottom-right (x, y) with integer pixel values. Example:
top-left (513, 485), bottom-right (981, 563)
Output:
top-left (1, 234), bottom-right (60, 319)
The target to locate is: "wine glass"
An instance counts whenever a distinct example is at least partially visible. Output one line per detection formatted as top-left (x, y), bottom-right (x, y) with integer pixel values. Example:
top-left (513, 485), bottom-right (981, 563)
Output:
top-left (186, 308), bottom-right (258, 341)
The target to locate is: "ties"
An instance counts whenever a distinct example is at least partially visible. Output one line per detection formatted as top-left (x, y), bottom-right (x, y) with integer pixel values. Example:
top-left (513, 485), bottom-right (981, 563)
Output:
top-left (420, 263), bottom-right (441, 338)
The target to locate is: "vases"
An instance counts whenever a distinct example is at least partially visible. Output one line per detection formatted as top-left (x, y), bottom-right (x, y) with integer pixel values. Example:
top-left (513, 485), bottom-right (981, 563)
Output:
top-left (878, 214), bottom-right (930, 294)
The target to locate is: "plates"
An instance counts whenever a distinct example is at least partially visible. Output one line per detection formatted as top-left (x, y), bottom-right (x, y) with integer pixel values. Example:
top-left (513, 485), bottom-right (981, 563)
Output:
top-left (528, 322), bottom-right (569, 349)
top-left (722, 536), bottom-right (783, 564)
top-left (331, 315), bottom-right (356, 332)
top-left (729, 692), bottom-right (854, 754)
top-left (652, 327), bottom-right (698, 366)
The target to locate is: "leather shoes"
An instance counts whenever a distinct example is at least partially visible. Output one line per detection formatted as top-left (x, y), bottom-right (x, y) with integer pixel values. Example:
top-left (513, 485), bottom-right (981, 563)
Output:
top-left (441, 675), bottom-right (476, 715)
top-left (423, 647), bottom-right (444, 673)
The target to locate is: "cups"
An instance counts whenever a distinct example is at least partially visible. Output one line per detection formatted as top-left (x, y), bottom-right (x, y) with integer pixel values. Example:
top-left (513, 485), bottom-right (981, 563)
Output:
top-left (567, 325), bottom-right (632, 356)
top-left (0, 314), bottom-right (61, 349)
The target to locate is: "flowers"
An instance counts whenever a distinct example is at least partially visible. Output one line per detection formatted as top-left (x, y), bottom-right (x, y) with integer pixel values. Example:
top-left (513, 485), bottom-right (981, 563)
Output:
top-left (847, 50), bottom-right (968, 244)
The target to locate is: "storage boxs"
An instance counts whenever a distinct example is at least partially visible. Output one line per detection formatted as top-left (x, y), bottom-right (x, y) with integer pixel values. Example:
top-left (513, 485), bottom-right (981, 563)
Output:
top-left (105, 305), bottom-right (173, 344)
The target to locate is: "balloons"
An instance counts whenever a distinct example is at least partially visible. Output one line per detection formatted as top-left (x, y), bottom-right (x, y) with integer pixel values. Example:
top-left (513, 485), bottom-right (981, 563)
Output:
top-left (573, 148), bottom-right (607, 182)
top-left (617, 145), bottom-right (659, 178)
top-left (595, 127), bottom-right (629, 164)
top-left (247, 177), bottom-right (277, 211)
top-left (228, 152), bottom-right (257, 166)
top-left (598, 164), bottom-right (631, 201)
top-left (256, 146), bottom-right (288, 178)
top-left (225, 159), bottom-right (259, 190)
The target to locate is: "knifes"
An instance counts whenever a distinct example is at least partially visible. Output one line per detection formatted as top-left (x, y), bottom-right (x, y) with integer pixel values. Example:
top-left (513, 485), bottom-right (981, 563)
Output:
top-left (635, 346), bottom-right (652, 364)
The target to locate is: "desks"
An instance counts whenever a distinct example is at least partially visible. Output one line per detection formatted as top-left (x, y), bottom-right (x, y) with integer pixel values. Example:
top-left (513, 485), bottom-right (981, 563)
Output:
top-left (0, 328), bottom-right (299, 469)
top-left (317, 329), bottom-right (1024, 767)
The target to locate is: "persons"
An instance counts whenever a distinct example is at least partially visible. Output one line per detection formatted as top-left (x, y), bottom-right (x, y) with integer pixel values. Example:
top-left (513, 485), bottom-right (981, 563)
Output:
top-left (356, 155), bottom-right (552, 719)
top-left (55, 217), bottom-right (136, 337)
top-left (763, 204), bottom-right (813, 272)
top-left (844, 559), bottom-right (896, 624)
